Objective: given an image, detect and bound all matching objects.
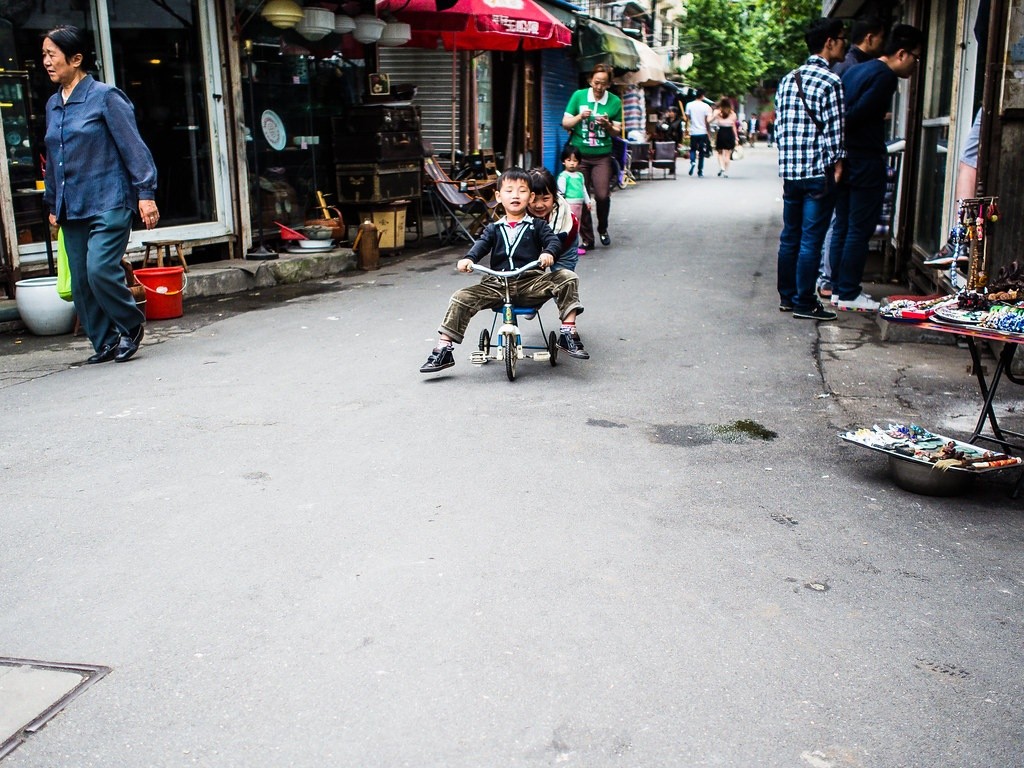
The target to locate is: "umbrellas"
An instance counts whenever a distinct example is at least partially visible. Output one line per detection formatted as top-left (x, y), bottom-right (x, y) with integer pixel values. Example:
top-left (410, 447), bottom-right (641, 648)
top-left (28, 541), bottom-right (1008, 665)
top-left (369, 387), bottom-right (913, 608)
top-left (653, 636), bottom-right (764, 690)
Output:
top-left (377, 1), bottom-right (574, 165)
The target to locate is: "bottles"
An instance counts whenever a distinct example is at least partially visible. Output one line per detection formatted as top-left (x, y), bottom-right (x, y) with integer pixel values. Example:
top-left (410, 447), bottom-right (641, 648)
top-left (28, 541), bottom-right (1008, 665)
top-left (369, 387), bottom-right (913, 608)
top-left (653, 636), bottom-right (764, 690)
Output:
top-left (471, 150), bottom-right (483, 179)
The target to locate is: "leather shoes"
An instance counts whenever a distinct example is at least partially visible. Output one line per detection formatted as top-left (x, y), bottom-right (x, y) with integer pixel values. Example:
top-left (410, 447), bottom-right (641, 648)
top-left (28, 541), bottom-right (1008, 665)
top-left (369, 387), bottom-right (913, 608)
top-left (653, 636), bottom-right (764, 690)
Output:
top-left (88, 344), bottom-right (118, 363)
top-left (115, 324), bottom-right (144, 362)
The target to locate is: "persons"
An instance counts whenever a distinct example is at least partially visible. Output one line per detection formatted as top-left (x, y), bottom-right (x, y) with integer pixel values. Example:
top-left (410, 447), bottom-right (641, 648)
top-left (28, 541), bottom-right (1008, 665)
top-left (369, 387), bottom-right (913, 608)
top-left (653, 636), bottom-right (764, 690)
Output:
top-left (923, 108), bottom-right (983, 270)
top-left (765, 118), bottom-right (774, 148)
top-left (420, 167), bottom-right (590, 372)
top-left (774, 18), bottom-right (922, 320)
top-left (562, 64), bottom-right (623, 251)
top-left (707, 97), bottom-right (738, 179)
top-left (656, 106), bottom-right (683, 176)
top-left (685, 89), bottom-right (712, 178)
top-left (527, 167), bottom-right (579, 273)
top-left (43, 26), bottom-right (160, 364)
top-left (556, 145), bottom-right (592, 255)
top-left (741, 113), bottom-right (760, 148)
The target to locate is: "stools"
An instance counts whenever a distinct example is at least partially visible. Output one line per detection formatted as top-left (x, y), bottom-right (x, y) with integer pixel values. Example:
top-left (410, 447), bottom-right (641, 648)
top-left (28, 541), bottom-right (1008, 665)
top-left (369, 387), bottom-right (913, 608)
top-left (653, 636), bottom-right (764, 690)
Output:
top-left (142, 241), bottom-right (189, 273)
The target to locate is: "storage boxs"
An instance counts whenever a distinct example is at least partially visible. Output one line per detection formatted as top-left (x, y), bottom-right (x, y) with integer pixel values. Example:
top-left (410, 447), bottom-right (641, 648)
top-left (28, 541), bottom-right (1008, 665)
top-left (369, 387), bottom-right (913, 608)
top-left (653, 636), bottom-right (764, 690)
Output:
top-left (331, 106), bottom-right (424, 204)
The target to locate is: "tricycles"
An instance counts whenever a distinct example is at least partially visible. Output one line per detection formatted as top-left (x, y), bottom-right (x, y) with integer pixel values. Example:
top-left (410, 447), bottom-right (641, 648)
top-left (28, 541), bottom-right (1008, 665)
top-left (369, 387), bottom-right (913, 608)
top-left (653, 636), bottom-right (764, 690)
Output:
top-left (454, 258), bottom-right (558, 382)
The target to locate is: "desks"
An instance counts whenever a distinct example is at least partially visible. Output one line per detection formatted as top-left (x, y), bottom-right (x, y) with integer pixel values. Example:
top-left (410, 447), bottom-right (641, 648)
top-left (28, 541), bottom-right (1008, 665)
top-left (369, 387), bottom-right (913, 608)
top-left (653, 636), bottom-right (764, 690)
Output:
top-left (889, 320), bottom-right (1024, 469)
top-left (462, 179), bottom-right (501, 236)
top-left (13, 192), bottom-right (55, 276)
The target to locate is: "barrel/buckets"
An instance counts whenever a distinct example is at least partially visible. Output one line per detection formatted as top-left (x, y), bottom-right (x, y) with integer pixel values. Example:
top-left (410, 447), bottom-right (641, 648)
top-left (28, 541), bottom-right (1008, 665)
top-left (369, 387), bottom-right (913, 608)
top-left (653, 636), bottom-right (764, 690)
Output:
top-left (135, 300), bottom-right (147, 327)
top-left (15, 277), bottom-right (77, 335)
top-left (133, 267), bottom-right (187, 319)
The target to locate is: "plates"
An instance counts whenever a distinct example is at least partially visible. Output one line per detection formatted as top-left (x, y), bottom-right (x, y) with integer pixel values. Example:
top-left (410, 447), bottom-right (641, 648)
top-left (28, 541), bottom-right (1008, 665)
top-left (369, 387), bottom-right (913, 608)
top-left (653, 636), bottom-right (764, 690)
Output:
top-left (930, 303), bottom-right (983, 329)
top-left (261, 110), bottom-right (287, 151)
top-left (287, 246), bottom-right (336, 253)
top-left (32, 190), bottom-right (45, 193)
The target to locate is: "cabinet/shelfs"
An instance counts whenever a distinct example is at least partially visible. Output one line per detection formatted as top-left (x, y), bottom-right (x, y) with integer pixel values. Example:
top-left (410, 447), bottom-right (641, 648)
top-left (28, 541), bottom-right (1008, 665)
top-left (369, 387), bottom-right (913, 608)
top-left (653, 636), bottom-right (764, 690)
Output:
top-left (0, 68), bottom-right (44, 192)
top-left (239, 40), bottom-right (319, 247)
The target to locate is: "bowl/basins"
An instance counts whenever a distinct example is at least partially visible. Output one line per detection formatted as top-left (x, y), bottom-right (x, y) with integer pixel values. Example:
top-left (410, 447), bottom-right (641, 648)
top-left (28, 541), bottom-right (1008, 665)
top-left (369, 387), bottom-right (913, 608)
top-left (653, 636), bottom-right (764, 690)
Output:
top-left (596, 116), bottom-right (607, 123)
top-left (305, 228), bottom-right (332, 240)
top-left (298, 239), bottom-right (334, 248)
top-left (382, 85), bottom-right (417, 102)
top-left (888, 455), bottom-right (968, 497)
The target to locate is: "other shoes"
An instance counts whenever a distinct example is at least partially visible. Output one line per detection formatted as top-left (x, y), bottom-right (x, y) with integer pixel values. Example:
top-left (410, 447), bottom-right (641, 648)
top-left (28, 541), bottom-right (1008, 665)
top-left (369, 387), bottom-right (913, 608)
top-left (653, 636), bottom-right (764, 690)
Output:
top-left (718, 170), bottom-right (723, 177)
top-left (600, 231), bottom-right (610, 245)
top-left (699, 174), bottom-right (703, 177)
top-left (578, 248), bottom-right (587, 255)
top-left (578, 242), bottom-right (594, 250)
top-left (689, 164), bottom-right (695, 175)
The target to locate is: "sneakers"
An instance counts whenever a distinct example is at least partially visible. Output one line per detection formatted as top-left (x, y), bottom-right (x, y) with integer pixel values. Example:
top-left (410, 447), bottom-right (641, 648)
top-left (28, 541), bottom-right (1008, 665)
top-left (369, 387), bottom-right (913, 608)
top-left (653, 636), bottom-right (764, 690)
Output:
top-left (923, 240), bottom-right (972, 269)
top-left (779, 302), bottom-right (795, 310)
top-left (421, 346), bottom-right (455, 372)
top-left (792, 307), bottom-right (837, 320)
top-left (838, 293), bottom-right (880, 312)
top-left (555, 330), bottom-right (590, 359)
top-left (830, 293), bottom-right (872, 307)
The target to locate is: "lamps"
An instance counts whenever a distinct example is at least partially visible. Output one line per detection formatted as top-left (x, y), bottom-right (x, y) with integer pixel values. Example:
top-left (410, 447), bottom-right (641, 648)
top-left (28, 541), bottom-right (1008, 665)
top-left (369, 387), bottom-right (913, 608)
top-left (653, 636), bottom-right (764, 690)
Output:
top-left (261, 0), bottom-right (411, 47)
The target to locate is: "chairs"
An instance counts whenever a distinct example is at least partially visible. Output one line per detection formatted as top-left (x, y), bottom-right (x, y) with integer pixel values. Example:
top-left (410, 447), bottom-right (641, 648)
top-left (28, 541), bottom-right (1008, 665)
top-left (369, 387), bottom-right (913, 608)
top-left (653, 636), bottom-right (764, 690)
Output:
top-left (652, 141), bottom-right (676, 179)
top-left (631, 144), bottom-right (651, 179)
top-left (480, 149), bottom-right (498, 182)
top-left (421, 140), bottom-right (498, 248)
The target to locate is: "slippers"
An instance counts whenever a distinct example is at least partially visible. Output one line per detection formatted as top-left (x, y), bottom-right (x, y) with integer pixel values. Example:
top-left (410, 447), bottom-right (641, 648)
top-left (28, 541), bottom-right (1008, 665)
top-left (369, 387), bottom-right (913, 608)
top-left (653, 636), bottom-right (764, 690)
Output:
top-left (817, 286), bottom-right (832, 298)
top-left (894, 443), bottom-right (938, 454)
top-left (914, 422), bottom-right (939, 442)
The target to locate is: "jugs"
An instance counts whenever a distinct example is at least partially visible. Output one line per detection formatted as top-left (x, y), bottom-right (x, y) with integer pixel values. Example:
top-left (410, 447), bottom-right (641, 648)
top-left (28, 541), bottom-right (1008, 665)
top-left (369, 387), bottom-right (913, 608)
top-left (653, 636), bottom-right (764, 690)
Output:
top-left (353, 218), bottom-right (382, 270)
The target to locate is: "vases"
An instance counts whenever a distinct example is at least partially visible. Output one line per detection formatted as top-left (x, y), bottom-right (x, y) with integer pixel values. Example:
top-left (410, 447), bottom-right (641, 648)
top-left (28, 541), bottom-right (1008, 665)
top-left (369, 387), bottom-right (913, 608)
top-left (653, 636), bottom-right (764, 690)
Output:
top-left (16, 276), bottom-right (77, 335)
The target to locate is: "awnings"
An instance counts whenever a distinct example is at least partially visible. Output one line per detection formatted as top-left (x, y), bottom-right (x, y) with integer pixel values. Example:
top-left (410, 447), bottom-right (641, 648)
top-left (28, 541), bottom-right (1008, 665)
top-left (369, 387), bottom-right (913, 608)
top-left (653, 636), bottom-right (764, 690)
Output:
top-left (575, 19), bottom-right (666, 87)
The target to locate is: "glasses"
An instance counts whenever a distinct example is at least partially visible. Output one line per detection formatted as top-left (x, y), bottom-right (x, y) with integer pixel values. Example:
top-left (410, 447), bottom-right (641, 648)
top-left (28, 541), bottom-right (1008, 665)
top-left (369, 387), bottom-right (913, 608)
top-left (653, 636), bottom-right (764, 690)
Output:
top-left (835, 34), bottom-right (848, 40)
top-left (904, 51), bottom-right (921, 62)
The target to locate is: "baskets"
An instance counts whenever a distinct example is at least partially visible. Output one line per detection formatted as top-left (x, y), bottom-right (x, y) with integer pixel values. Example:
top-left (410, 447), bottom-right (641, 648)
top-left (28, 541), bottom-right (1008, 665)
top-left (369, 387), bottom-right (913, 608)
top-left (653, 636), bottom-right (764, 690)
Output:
top-left (302, 206), bottom-right (346, 237)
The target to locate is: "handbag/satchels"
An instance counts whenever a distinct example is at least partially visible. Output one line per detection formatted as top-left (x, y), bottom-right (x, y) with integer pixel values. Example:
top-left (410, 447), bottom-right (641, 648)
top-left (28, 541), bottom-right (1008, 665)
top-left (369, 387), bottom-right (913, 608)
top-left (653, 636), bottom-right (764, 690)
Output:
top-left (730, 144), bottom-right (745, 161)
top-left (701, 139), bottom-right (713, 157)
top-left (55, 225), bottom-right (73, 302)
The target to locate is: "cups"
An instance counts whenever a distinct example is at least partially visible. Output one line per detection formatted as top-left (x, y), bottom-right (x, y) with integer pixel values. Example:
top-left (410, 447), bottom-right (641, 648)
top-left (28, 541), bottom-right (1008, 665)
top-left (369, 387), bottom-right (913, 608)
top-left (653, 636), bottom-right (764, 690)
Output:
top-left (294, 137), bottom-right (319, 145)
top-left (36, 181), bottom-right (45, 190)
